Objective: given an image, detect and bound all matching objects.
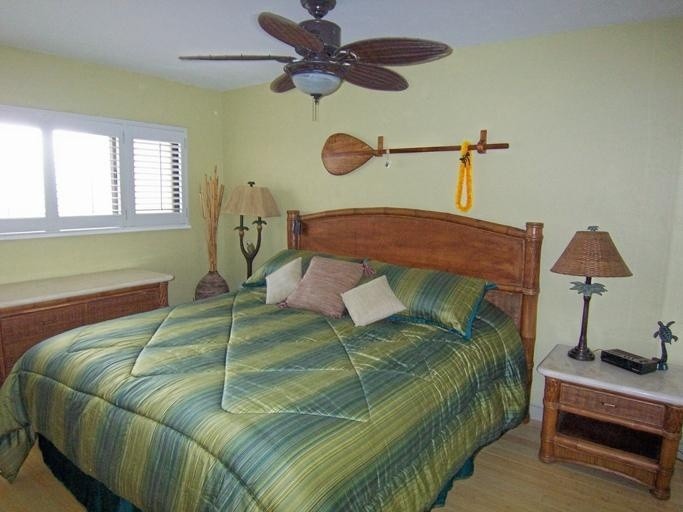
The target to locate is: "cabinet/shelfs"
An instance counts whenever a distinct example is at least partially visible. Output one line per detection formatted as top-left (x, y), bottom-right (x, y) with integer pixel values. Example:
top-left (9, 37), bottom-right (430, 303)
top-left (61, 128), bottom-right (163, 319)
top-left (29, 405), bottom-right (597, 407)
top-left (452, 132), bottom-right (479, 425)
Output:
top-left (0, 268), bottom-right (175, 386)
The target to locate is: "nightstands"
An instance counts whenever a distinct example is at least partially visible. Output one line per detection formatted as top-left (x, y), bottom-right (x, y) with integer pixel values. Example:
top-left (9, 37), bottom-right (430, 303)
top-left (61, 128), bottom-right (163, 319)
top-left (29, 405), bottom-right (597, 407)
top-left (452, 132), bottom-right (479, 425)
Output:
top-left (536, 344), bottom-right (682, 501)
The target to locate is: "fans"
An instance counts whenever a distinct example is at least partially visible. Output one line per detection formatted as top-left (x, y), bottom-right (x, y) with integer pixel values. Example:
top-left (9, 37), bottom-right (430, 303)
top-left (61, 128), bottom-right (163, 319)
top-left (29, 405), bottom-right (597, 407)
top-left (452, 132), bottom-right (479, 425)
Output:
top-left (177, 0), bottom-right (453, 120)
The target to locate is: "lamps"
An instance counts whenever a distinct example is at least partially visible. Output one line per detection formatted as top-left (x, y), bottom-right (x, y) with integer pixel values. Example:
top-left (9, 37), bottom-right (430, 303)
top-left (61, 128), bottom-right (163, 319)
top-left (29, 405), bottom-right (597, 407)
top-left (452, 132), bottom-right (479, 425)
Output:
top-left (282, 59), bottom-right (352, 106)
top-left (221, 182), bottom-right (282, 280)
top-left (550, 225), bottom-right (634, 361)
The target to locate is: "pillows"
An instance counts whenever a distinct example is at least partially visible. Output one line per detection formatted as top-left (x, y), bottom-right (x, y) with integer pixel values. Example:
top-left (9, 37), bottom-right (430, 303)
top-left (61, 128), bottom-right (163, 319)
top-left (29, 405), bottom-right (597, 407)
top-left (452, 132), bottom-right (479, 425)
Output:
top-left (241, 249), bottom-right (498, 341)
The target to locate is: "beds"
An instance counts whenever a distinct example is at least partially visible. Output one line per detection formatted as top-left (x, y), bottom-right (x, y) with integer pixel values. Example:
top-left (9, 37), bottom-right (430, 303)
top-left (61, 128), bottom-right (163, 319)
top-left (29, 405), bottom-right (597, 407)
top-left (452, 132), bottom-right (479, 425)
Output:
top-left (0, 209), bottom-right (544, 512)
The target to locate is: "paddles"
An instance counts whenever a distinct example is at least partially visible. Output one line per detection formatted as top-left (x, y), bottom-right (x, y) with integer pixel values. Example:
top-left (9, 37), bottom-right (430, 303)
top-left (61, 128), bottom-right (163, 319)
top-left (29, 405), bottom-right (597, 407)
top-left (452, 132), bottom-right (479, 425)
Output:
top-left (321, 133), bottom-right (509, 175)
